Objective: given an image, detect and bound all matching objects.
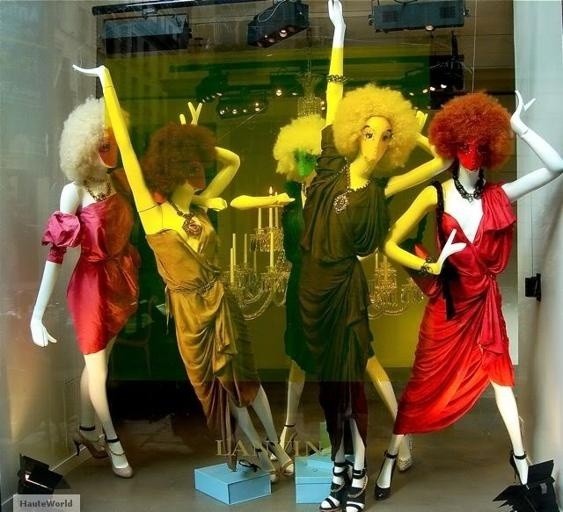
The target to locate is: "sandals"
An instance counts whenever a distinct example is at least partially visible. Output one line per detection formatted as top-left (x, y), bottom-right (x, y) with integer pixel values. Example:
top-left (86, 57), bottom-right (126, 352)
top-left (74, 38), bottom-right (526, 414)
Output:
top-left (253, 438), bottom-right (295, 485)
top-left (319, 460), bottom-right (349, 512)
top-left (342, 469), bottom-right (369, 512)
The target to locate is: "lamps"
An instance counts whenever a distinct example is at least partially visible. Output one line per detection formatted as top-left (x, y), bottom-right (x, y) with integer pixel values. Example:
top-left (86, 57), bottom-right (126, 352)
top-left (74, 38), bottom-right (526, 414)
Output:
top-left (99, 6), bottom-right (192, 56)
top-left (367, 0), bottom-right (467, 32)
top-left (245, 1), bottom-right (308, 48)
top-left (191, 65), bottom-right (305, 120)
top-left (398, 59), bottom-right (465, 110)
top-left (17, 453), bottom-right (63, 494)
top-left (492, 459), bottom-right (560, 512)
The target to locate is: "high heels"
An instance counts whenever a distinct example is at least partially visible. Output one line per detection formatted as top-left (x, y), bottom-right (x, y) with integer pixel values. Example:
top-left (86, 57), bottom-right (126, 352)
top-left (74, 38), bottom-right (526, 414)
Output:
top-left (73, 426), bottom-right (109, 459)
top-left (509, 450), bottom-right (529, 485)
top-left (270, 424), bottom-right (297, 461)
top-left (104, 436), bottom-right (134, 478)
top-left (395, 449), bottom-right (413, 472)
top-left (374, 449), bottom-right (398, 501)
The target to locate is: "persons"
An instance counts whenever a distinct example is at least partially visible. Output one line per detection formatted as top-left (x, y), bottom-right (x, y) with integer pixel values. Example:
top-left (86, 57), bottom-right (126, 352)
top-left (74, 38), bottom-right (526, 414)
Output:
top-left (27, 1), bottom-right (563, 512)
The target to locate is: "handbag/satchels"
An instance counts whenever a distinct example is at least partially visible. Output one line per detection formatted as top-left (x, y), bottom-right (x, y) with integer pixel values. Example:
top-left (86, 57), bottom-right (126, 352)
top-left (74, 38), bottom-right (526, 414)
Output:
top-left (397, 238), bottom-right (448, 298)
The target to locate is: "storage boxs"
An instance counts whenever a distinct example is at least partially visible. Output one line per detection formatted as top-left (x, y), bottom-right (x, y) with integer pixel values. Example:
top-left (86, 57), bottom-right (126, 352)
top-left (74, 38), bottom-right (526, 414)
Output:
top-left (295, 454), bottom-right (354, 504)
top-left (194, 461), bottom-right (271, 506)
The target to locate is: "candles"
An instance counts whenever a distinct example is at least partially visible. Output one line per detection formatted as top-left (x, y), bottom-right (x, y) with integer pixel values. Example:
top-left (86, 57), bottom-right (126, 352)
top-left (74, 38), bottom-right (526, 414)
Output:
top-left (375, 253), bottom-right (387, 270)
top-left (229, 185), bottom-right (278, 285)
